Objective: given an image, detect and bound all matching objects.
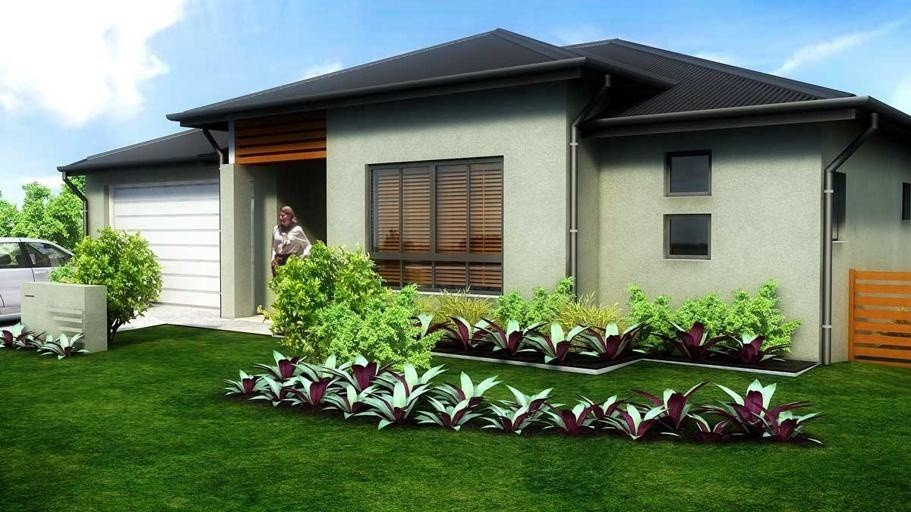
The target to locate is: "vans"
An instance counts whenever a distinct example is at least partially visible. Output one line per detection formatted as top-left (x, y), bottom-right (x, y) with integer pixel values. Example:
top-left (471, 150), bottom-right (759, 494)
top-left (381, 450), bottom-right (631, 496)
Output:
top-left (0, 237), bottom-right (80, 322)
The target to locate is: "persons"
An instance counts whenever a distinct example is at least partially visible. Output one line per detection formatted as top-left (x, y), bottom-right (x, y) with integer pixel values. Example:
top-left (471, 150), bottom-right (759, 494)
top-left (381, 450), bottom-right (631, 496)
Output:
top-left (268, 205), bottom-right (313, 336)
